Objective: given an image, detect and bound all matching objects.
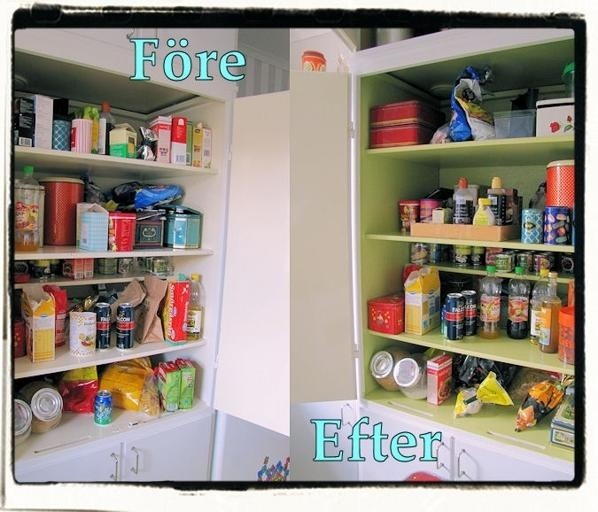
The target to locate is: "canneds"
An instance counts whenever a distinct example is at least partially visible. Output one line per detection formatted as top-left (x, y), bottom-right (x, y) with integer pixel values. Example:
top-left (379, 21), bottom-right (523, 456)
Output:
top-left (462, 290), bottom-right (477, 336)
top-left (115, 302), bottom-right (134, 349)
top-left (95, 389), bottom-right (112, 426)
top-left (94, 302), bottom-right (110, 349)
top-left (441, 292), bottom-right (465, 340)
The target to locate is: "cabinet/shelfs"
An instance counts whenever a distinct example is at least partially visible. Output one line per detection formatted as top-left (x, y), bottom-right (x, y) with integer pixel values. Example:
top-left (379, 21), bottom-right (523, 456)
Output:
top-left (347, 28), bottom-right (575, 482)
top-left (15, 28), bottom-right (240, 482)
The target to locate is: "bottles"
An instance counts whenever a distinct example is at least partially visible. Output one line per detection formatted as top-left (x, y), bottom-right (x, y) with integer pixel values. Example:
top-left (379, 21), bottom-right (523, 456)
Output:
top-left (14, 164), bottom-right (41, 252)
top-left (530, 269), bottom-right (551, 345)
top-left (479, 264), bottom-right (502, 340)
top-left (507, 266), bottom-right (531, 339)
top-left (187, 274), bottom-right (203, 340)
top-left (539, 271), bottom-right (562, 354)
top-left (471, 198), bottom-right (497, 267)
top-left (488, 177), bottom-right (507, 225)
top-left (452, 178), bottom-right (474, 268)
